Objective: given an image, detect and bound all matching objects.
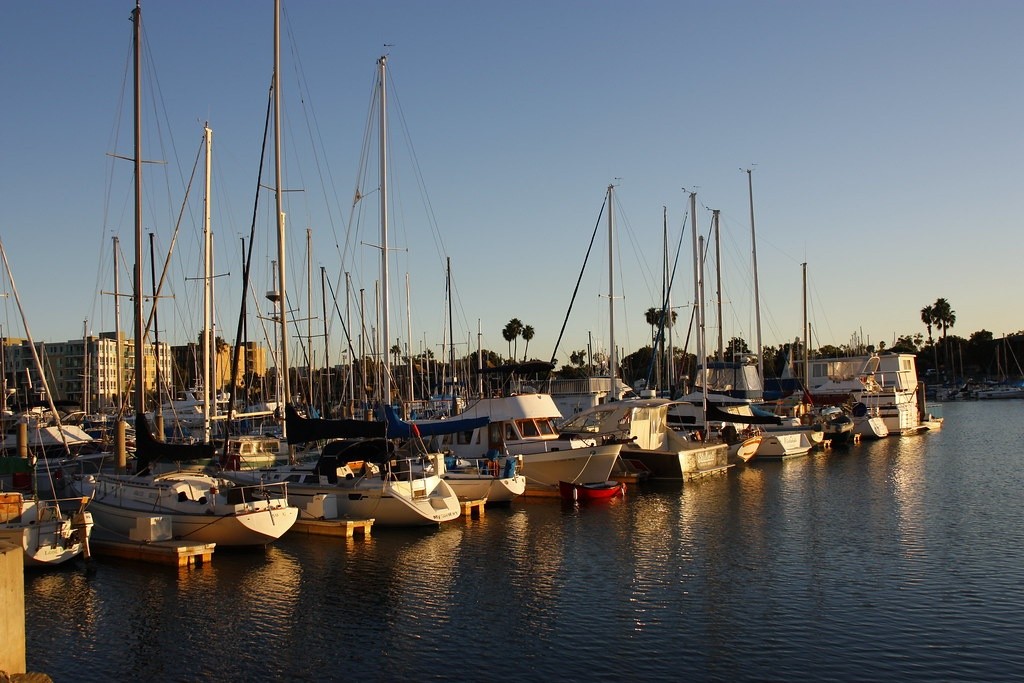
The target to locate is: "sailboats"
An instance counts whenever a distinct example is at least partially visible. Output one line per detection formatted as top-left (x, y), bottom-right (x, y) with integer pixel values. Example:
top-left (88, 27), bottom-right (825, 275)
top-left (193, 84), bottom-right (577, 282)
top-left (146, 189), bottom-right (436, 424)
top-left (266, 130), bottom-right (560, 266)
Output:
top-left (944, 334), bottom-right (1024, 399)
top-left (10, 0), bottom-right (943, 565)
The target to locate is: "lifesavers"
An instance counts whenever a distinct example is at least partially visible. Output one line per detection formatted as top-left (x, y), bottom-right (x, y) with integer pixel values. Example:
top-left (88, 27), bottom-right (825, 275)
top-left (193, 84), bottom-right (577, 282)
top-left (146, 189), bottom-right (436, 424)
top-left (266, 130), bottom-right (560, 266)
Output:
top-left (800, 414), bottom-right (813, 427)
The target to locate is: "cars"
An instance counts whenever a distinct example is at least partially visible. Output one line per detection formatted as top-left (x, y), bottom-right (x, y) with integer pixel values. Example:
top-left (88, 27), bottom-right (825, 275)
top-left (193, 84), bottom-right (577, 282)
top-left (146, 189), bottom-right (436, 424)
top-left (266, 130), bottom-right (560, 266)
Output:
top-left (926, 369), bottom-right (937, 375)
